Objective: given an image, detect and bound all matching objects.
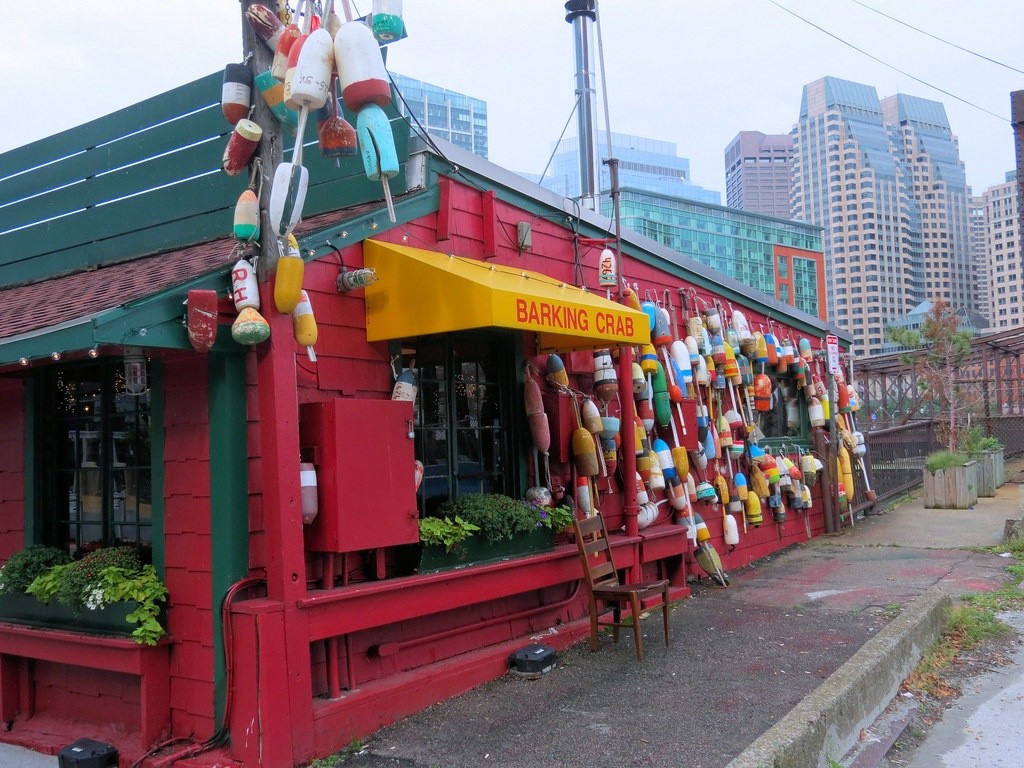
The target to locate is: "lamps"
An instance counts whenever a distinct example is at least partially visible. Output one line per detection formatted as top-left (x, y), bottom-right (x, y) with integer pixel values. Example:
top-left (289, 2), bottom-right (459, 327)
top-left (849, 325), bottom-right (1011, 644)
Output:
top-left (18, 353), bottom-right (32, 366)
top-left (87, 343), bottom-right (101, 359)
top-left (51, 350), bottom-right (66, 361)
top-left (336, 267), bottom-right (378, 294)
top-left (123, 347), bottom-right (147, 396)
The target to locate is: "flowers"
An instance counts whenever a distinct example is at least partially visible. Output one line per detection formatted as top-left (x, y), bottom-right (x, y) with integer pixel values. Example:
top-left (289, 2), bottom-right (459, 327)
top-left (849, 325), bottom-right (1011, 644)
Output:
top-left (0, 545), bottom-right (169, 647)
top-left (518, 497), bottom-right (548, 528)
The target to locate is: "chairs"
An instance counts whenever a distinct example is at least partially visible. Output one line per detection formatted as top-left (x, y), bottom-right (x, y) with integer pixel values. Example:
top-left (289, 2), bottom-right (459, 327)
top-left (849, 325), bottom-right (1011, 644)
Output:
top-left (572, 512), bottom-right (671, 661)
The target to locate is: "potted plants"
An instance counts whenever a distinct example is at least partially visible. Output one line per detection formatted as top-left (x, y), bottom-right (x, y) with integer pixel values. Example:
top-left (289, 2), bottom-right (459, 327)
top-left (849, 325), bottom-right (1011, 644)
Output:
top-left (884, 301), bottom-right (1005, 510)
top-left (418, 492), bottom-right (572, 575)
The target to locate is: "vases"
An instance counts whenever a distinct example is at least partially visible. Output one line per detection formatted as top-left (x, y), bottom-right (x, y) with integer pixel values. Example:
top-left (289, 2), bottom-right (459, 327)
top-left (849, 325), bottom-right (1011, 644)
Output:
top-left (0, 595), bottom-right (165, 637)
